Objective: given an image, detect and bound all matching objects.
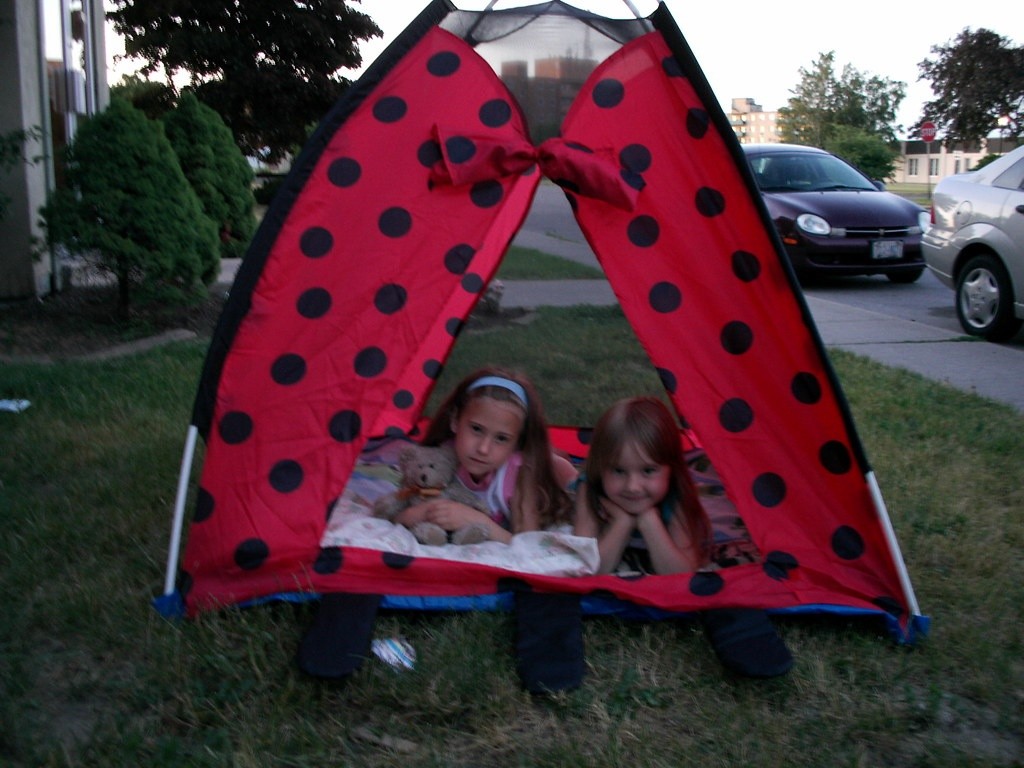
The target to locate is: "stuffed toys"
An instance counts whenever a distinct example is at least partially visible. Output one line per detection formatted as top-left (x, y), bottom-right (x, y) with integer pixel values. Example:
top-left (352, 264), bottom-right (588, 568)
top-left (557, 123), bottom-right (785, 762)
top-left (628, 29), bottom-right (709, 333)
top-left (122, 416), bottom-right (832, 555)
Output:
top-left (375, 440), bottom-right (487, 548)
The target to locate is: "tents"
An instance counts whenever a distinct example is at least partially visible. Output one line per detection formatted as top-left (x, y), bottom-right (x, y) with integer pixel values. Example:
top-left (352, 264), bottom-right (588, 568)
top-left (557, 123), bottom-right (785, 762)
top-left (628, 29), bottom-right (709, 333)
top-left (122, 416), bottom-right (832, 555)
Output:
top-left (150, 0), bottom-right (928, 647)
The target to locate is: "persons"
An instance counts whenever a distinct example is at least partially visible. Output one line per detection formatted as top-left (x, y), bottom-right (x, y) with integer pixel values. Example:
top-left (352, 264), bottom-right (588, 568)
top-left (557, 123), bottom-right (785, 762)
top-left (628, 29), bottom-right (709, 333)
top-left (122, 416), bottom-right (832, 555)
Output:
top-left (391, 365), bottom-right (582, 546)
top-left (544, 395), bottom-right (710, 578)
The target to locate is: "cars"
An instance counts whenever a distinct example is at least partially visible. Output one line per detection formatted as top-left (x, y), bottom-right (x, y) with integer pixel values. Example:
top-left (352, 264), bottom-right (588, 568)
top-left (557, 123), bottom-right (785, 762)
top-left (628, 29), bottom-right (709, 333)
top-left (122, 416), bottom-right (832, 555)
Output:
top-left (920, 122), bottom-right (1024, 341)
top-left (742, 141), bottom-right (932, 285)
